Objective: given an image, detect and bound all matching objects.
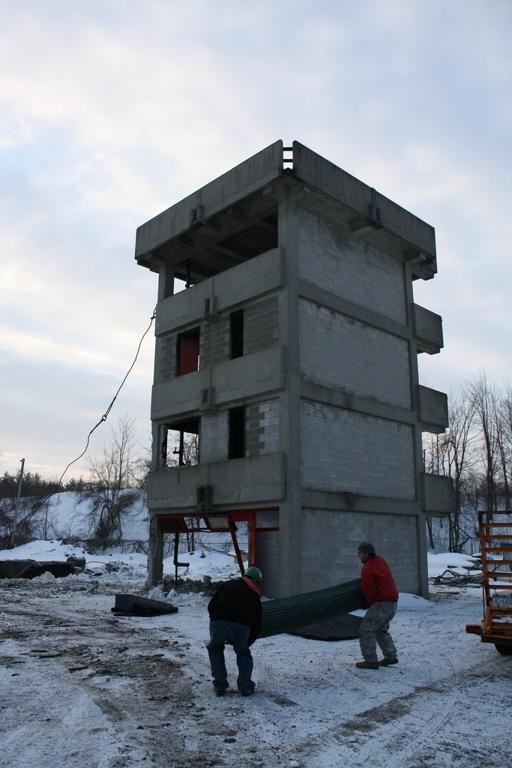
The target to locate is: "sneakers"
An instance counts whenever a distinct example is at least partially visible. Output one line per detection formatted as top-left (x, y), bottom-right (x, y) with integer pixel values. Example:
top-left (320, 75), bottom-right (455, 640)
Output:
top-left (240, 687), bottom-right (254, 697)
top-left (215, 688), bottom-right (226, 696)
top-left (356, 657), bottom-right (398, 669)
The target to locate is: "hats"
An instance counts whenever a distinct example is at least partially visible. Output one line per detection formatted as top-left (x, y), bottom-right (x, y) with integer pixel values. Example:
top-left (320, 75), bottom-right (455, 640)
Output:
top-left (245, 566), bottom-right (262, 582)
top-left (357, 542), bottom-right (375, 553)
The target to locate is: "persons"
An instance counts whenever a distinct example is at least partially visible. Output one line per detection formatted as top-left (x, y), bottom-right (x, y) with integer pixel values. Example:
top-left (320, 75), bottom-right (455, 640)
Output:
top-left (206, 565), bottom-right (263, 696)
top-left (354, 541), bottom-right (399, 669)
